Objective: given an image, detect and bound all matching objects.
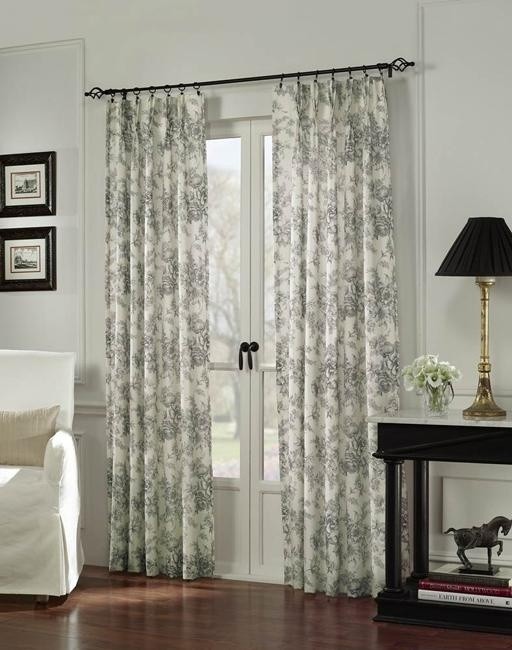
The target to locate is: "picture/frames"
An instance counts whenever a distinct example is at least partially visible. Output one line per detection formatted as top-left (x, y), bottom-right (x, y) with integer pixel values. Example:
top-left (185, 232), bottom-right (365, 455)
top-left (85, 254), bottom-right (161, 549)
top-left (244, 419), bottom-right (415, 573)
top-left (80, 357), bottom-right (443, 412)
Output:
top-left (0, 228), bottom-right (56, 290)
top-left (0, 152), bottom-right (56, 217)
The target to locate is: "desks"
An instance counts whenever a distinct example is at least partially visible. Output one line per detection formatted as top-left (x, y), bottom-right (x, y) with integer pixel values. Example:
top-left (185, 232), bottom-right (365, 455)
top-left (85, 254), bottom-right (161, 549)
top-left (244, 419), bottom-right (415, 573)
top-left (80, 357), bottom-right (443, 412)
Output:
top-left (372, 407), bottom-right (511, 633)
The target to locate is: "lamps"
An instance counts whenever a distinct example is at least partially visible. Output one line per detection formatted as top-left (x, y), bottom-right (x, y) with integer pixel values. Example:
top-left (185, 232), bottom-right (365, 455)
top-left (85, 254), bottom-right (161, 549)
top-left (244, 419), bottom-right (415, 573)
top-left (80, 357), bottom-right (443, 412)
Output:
top-left (433, 218), bottom-right (512, 419)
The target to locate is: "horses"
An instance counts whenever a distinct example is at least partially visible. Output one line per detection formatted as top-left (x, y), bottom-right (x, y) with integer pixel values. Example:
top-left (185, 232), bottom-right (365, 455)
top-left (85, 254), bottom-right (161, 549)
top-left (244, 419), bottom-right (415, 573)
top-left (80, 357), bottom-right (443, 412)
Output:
top-left (443, 515), bottom-right (512, 570)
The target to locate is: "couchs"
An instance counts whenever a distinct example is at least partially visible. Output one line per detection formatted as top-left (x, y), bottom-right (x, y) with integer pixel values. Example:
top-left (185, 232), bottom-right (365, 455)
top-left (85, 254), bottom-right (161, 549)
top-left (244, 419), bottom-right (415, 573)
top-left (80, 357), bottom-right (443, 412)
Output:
top-left (0, 348), bottom-right (82, 605)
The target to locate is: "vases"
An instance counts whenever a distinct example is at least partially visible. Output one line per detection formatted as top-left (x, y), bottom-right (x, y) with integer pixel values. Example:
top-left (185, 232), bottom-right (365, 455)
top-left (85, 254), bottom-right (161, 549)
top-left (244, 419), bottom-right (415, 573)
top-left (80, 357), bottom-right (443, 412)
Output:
top-left (424, 383), bottom-right (454, 417)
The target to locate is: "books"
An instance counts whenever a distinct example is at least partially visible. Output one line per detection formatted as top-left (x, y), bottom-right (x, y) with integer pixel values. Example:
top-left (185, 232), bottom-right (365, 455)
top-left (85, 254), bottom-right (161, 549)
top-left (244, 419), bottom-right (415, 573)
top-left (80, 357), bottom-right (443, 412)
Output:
top-left (417, 563), bottom-right (512, 608)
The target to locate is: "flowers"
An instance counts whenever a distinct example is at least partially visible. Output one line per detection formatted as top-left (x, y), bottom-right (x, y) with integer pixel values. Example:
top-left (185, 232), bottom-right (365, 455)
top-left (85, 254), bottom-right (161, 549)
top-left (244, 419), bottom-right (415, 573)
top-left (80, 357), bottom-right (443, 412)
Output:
top-left (400, 354), bottom-right (460, 394)
